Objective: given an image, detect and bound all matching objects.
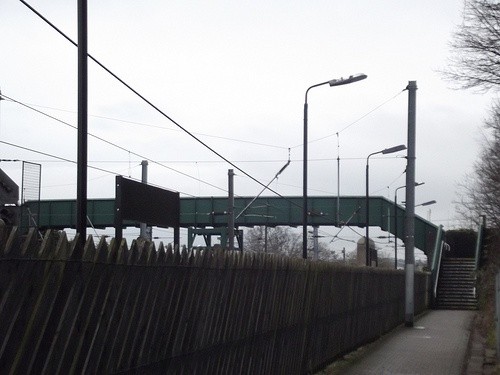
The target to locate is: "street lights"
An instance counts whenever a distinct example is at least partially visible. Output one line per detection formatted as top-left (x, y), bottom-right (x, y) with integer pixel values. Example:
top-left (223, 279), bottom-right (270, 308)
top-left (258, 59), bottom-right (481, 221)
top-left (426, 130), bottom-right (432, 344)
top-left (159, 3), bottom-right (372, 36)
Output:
top-left (394, 181), bottom-right (426, 268)
top-left (302, 73), bottom-right (367, 259)
top-left (415, 200), bottom-right (437, 208)
top-left (365, 145), bottom-right (407, 267)
